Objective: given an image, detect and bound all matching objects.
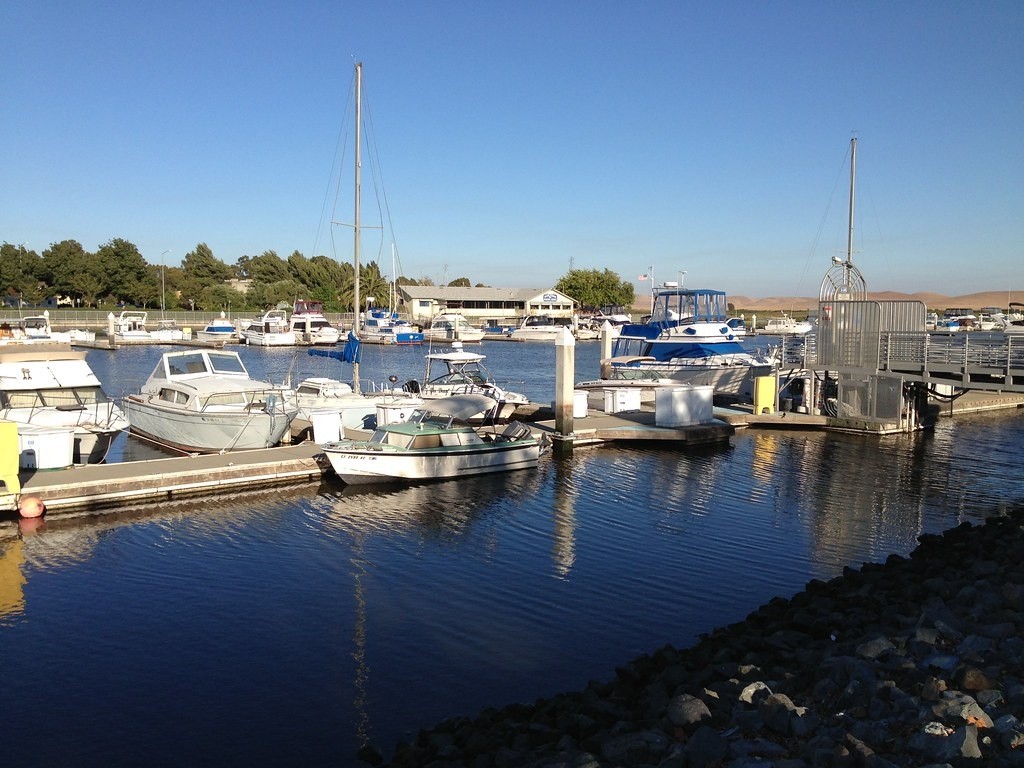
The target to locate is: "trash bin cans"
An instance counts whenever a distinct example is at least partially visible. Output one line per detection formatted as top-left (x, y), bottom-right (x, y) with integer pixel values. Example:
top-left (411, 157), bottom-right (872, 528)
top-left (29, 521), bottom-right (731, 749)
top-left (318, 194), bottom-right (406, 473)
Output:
top-left (310, 408), bottom-right (343, 443)
top-left (574, 391), bottom-right (589, 419)
top-left (20, 427), bottom-right (75, 470)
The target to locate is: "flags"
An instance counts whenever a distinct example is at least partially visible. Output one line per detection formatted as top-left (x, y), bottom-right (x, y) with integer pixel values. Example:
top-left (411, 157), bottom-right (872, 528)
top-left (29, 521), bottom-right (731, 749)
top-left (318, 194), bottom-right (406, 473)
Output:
top-left (639, 274), bottom-right (647, 280)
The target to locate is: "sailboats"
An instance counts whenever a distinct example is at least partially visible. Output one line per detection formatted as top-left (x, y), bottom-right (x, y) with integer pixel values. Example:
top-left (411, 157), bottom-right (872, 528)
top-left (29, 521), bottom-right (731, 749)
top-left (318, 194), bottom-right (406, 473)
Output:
top-left (283, 61), bottom-right (533, 438)
top-left (778, 134), bottom-right (969, 414)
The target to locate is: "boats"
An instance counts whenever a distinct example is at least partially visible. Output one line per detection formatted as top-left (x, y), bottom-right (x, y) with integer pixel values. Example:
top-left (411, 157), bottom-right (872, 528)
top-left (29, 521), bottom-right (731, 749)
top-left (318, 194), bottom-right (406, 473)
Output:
top-left (240, 309), bottom-right (295, 347)
top-left (612, 276), bottom-right (780, 398)
top-left (122, 345), bottom-right (302, 454)
top-left (0, 341), bottom-right (130, 464)
top-left (421, 312), bottom-right (485, 344)
top-left (511, 315), bottom-right (562, 340)
top-left (765, 317), bottom-right (812, 335)
top-left (320, 395), bottom-right (539, 483)
top-left (287, 312), bottom-right (340, 345)
top-left (102, 310), bottom-right (151, 339)
top-left (361, 307), bottom-right (423, 345)
top-left (196, 318), bottom-right (241, 344)
